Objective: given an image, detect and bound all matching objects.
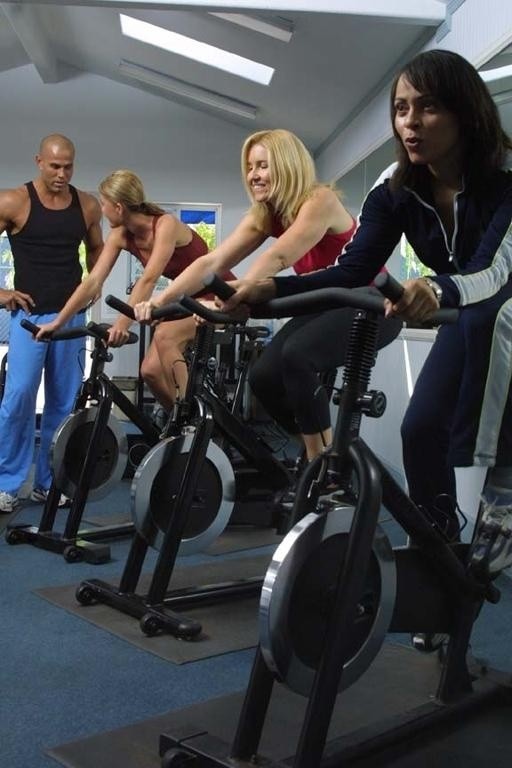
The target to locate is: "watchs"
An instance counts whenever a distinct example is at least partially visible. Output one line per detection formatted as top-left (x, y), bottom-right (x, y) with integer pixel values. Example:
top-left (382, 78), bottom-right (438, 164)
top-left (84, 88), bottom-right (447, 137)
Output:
top-left (419, 276), bottom-right (444, 304)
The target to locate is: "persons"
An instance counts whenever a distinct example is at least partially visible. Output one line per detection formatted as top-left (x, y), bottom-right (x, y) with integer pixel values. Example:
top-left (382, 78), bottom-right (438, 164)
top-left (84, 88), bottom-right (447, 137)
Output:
top-left (26, 167), bottom-right (236, 428)
top-left (0, 130), bottom-right (106, 514)
top-left (132, 127), bottom-right (405, 512)
top-left (212, 47), bottom-right (512, 648)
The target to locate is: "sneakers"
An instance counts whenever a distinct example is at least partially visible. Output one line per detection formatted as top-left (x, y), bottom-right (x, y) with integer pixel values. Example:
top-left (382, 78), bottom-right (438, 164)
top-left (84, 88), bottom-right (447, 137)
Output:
top-left (410, 629), bottom-right (452, 653)
top-left (470, 487), bottom-right (512, 575)
top-left (282, 466), bottom-right (344, 509)
top-left (0, 491), bottom-right (22, 513)
top-left (31, 487), bottom-right (74, 508)
